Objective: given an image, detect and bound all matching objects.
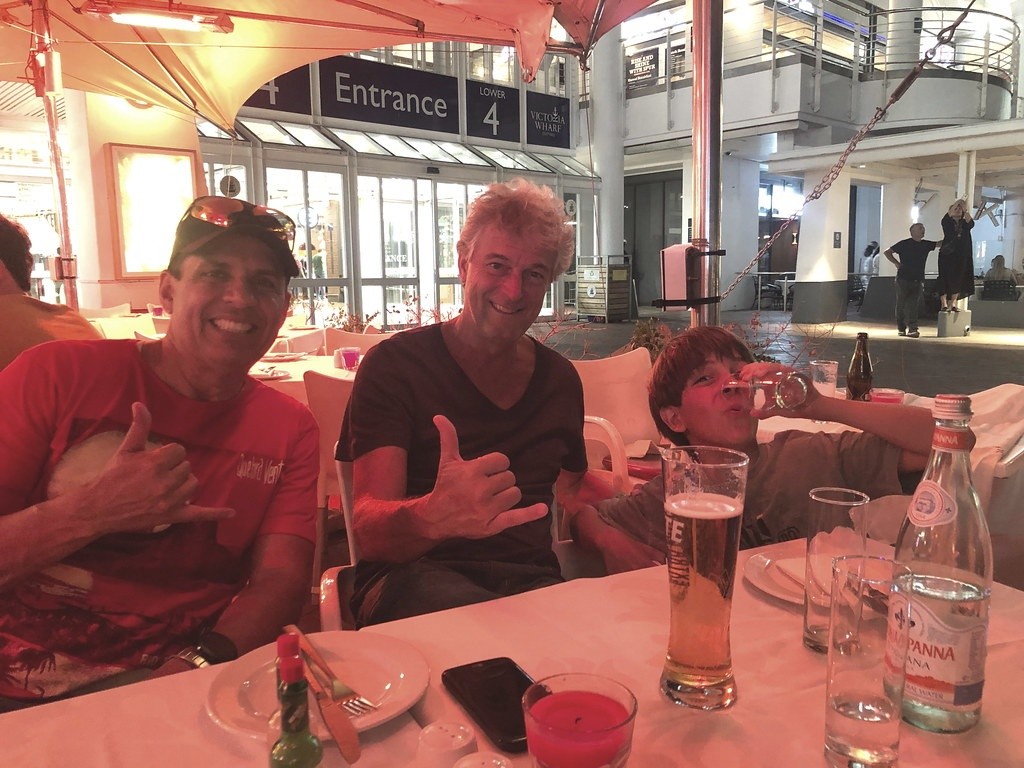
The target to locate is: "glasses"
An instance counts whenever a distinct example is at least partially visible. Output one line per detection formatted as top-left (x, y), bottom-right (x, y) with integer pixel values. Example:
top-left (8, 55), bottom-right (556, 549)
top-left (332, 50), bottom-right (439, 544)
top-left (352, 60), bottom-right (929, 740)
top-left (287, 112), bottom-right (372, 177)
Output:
top-left (175, 195), bottom-right (296, 261)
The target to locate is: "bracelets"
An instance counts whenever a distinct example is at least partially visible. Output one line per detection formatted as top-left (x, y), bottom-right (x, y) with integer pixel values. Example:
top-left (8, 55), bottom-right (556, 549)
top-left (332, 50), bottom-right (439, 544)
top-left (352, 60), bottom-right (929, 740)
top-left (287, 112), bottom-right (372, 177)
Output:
top-left (175, 648), bottom-right (210, 669)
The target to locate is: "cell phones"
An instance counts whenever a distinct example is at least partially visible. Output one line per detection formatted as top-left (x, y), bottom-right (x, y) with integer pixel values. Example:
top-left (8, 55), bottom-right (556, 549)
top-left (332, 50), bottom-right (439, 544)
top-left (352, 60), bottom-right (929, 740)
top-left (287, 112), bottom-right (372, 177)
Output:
top-left (961, 193), bottom-right (968, 202)
top-left (441, 656), bottom-right (550, 753)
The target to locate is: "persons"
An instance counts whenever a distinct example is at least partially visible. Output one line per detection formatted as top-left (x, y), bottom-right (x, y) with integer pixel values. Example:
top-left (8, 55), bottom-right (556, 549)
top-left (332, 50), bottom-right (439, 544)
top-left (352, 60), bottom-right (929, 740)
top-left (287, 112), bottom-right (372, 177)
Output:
top-left (938, 199), bottom-right (974, 313)
top-left (0, 197), bottom-right (322, 712)
top-left (860, 241), bottom-right (880, 294)
top-left (0, 214), bottom-right (109, 369)
top-left (978, 268), bottom-right (985, 280)
top-left (299, 248), bottom-right (328, 300)
top-left (883, 223), bottom-right (943, 338)
top-left (291, 254), bottom-right (303, 304)
top-left (335, 178), bottom-right (586, 628)
top-left (50, 247), bottom-right (65, 304)
top-left (1010, 257), bottom-right (1024, 285)
top-left (984, 255), bottom-right (1018, 294)
top-left (570, 325), bottom-right (976, 571)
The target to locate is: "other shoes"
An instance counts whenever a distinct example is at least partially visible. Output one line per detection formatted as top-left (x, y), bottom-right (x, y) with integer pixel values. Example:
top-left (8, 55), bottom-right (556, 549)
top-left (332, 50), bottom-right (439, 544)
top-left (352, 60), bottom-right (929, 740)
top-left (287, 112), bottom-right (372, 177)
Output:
top-left (941, 307), bottom-right (951, 312)
top-left (898, 331), bottom-right (906, 335)
top-left (908, 331), bottom-right (920, 337)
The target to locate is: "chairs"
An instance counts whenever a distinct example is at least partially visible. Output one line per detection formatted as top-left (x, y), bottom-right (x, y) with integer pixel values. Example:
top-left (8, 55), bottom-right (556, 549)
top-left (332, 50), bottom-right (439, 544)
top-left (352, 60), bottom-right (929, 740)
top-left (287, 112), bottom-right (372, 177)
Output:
top-left (983, 278), bottom-right (1021, 301)
top-left (847, 275), bottom-right (865, 312)
top-left (921, 288), bottom-right (940, 319)
top-left (752, 277), bottom-right (796, 311)
top-left (76, 303), bottom-right (662, 632)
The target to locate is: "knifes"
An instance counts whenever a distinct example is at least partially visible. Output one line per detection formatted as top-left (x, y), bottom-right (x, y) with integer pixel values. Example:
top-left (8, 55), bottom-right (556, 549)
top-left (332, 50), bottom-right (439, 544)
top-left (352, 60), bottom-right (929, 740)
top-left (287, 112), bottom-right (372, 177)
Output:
top-left (832, 566), bottom-right (890, 616)
top-left (300, 650), bottom-right (361, 765)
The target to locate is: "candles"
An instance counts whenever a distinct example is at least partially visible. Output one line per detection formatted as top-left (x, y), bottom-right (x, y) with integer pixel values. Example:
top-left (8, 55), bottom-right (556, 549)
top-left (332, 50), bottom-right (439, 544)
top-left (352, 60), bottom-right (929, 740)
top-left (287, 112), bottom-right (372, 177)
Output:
top-left (527, 691), bottom-right (630, 768)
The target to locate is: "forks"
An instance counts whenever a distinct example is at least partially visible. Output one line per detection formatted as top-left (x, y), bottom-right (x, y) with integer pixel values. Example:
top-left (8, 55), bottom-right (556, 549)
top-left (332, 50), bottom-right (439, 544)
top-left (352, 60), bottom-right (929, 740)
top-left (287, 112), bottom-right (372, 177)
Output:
top-left (283, 624), bottom-right (379, 716)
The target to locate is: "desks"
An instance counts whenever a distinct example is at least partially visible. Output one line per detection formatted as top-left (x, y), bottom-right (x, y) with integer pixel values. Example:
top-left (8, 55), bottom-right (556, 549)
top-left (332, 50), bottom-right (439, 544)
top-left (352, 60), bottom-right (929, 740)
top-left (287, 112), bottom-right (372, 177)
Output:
top-left (602, 444), bottom-right (669, 481)
top-left (754, 388), bottom-right (1024, 593)
top-left (970, 284), bottom-right (1024, 302)
top-left (245, 354), bottom-right (375, 543)
top-left (0, 529), bottom-right (1024, 768)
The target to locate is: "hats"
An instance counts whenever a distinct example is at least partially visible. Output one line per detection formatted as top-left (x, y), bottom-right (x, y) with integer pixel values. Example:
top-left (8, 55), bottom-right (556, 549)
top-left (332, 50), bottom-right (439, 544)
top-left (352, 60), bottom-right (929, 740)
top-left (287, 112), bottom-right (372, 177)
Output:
top-left (177, 196), bottom-right (300, 278)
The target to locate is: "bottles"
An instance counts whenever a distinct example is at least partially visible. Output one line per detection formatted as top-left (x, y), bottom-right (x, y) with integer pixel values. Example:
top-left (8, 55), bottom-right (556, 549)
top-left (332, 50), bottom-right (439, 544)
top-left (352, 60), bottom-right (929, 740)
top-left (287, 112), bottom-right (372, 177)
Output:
top-left (883, 394), bottom-right (992, 735)
top-left (264, 632), bottom-right (324, 767)
top-left (846, 331), bottom-right (872, 402)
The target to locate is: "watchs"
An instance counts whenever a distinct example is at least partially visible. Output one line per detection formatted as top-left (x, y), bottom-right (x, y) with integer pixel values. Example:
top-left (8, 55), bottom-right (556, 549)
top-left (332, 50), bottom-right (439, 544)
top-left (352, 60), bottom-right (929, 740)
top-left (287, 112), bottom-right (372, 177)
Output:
top-left (184, 630), bottom-right (238, 664)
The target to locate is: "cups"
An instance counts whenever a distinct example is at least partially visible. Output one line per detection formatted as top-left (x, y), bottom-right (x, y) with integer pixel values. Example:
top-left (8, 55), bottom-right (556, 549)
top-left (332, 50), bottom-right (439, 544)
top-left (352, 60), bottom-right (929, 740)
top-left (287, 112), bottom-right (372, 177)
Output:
top-left (521, 672), bottom-right (639, 768)
top-left (721, 373), bottom-right (807, 412)
top-left (802, 488), bottom-right (870, 653)
top-left (417, 720), bottom-right (513, 768)
top-left (809, 360), bottom-right (839, 397)
top-left (152, 305), bottom-right (163, 317)
top-left (334, 350), bottom-right (343, 368)
top-left (339, 347), bottom-right (361, 370)
top-left (660, 446), bottom-right (749, 713)
top-left (823, 554), bottom-right (914, 768)
top-left (869, 388), bottom-right (904, 403)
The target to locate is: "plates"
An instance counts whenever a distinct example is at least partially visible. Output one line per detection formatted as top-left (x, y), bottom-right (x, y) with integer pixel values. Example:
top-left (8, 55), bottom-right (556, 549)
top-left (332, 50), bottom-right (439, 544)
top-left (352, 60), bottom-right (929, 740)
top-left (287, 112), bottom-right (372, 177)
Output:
top-left (259, 352), bottom-right (308, 362)
top-left (248, 369), bottom-right (290, 380)
top-left (203, 630), bottom-right (430, 742)
top-left (743, 548), bottom-right (893, 606)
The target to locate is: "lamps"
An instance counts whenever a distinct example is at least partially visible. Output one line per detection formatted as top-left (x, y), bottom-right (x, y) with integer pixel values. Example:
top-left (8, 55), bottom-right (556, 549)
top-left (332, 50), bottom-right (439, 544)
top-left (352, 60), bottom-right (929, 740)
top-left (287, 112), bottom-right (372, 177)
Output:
top-left (80, 0), bottom-right (234, 35)
top-left (44, 51), bottom-right (62, 95)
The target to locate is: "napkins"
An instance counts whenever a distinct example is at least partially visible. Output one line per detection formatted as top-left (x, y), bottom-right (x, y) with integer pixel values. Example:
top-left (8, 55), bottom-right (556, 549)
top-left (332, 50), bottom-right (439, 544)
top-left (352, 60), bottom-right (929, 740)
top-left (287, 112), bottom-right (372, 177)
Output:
top-left (777, 555), bottom-right (890, 620)
